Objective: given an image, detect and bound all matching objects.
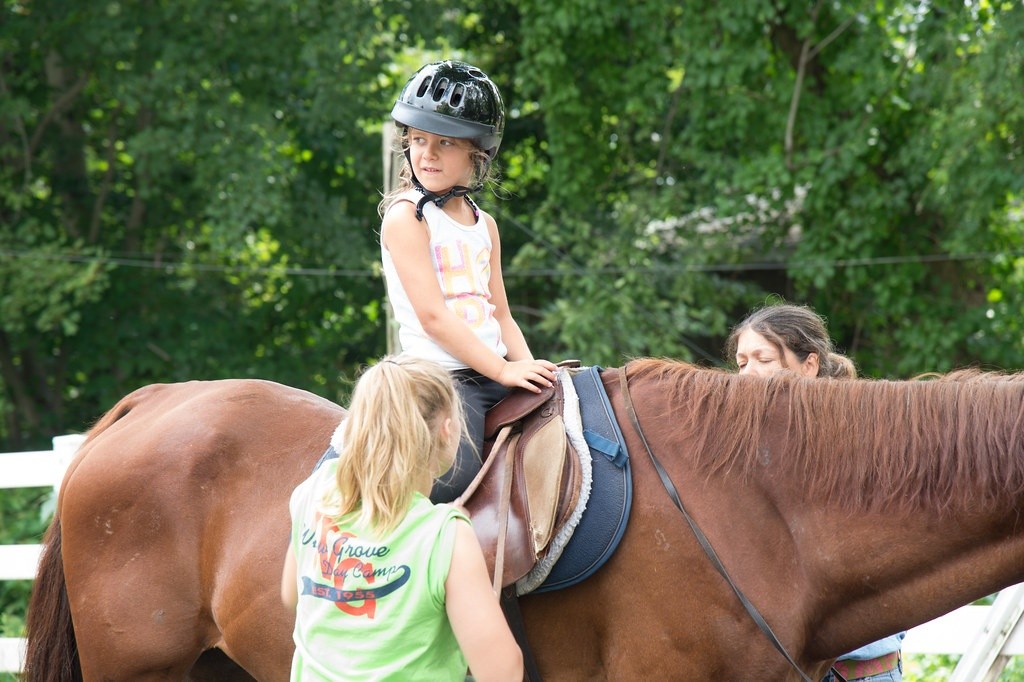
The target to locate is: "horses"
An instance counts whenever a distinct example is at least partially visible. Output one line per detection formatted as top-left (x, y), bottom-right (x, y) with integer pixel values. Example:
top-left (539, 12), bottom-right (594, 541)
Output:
top-left (20, 363), bottom-right (1024, 682)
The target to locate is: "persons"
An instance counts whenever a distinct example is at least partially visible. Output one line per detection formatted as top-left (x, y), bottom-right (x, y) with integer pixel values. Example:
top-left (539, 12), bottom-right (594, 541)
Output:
top-left (378, 58), bottom-right (558, 509)
top-left (278, 355), bottom-right (526, 682)
top-left (724, 303), bottom-right (907, 682)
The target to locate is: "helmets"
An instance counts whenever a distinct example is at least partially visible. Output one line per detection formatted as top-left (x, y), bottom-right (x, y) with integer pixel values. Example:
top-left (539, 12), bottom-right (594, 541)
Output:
top-left (390, 60), bottom-right (506, 160)
